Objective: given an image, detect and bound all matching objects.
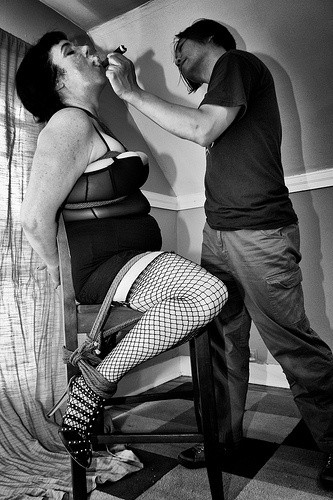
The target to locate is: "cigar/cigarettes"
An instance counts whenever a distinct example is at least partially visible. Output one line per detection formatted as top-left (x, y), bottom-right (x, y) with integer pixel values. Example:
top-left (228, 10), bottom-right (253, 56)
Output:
top-left (99, 44), bottom-right (127, 69)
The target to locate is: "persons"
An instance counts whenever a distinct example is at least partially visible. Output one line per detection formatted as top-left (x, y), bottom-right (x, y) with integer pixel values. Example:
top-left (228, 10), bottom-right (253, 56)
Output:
top-left (13, 29), bottom-right (230, 472)
top-left (106, 18), bottom-right (333, 492)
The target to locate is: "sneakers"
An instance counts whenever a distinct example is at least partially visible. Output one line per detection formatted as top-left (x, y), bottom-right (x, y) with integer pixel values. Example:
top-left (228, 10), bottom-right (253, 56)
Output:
top-left (317, 455), bottom-right (333, 492)
top-left (179, 440), bottom-right (245, 468)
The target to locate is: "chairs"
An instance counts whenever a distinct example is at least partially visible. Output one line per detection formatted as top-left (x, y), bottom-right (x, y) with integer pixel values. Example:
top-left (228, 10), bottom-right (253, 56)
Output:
top-left (55, 213), bottom-right (225, 499)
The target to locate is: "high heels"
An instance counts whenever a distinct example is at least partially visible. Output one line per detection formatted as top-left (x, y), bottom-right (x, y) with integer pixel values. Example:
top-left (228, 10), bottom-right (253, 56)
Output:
top-left (48, 376), bottom-right (106, 469)
